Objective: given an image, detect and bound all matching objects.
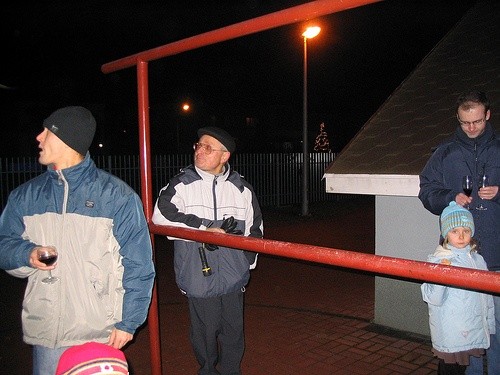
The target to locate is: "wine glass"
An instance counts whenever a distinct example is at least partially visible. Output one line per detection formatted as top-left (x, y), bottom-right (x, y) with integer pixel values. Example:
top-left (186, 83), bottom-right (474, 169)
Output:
top-left (462, 175), bottom-right (475, 209)
top-left (476, 175), bottom-right (490, 210)
top-left (36, 245), bottom-right (59, 283)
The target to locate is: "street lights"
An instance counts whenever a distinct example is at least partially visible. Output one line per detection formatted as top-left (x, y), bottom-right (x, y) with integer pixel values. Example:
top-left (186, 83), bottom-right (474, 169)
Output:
top-left (302, 26), bottom-right (321, 216)
top-left (175, 101), bottom-right (190, 153)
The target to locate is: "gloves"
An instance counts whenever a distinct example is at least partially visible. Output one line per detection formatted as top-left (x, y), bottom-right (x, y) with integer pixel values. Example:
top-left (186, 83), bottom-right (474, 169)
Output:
top-left (204, 216), bottom-right (243, 252)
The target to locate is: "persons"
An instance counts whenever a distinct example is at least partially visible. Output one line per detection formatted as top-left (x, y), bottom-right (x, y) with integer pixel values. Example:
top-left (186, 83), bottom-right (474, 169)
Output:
top-left (0, 106), bottom-right (156, 375)
top-left (151, 127), bottom-right (263, 375)
top-left (417, 90), bottom-right (500, 375)
top-left (421, 201), bottom-right (496, 375)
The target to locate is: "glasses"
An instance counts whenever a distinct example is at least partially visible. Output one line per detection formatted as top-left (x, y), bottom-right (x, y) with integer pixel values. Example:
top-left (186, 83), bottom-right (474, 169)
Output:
top-left (457, 115), bottom-right (485, 126)
top-left (193, 141), bottom-right (228, 154)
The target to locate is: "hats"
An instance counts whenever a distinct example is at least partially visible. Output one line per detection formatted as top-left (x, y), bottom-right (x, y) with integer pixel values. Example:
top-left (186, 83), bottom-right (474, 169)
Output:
top-left (197, 126), bottom-right (239, 154)
top-left (44, 107), bottom-right (97, 158)
top-left (441, 200), bottom-right (475, 239)
